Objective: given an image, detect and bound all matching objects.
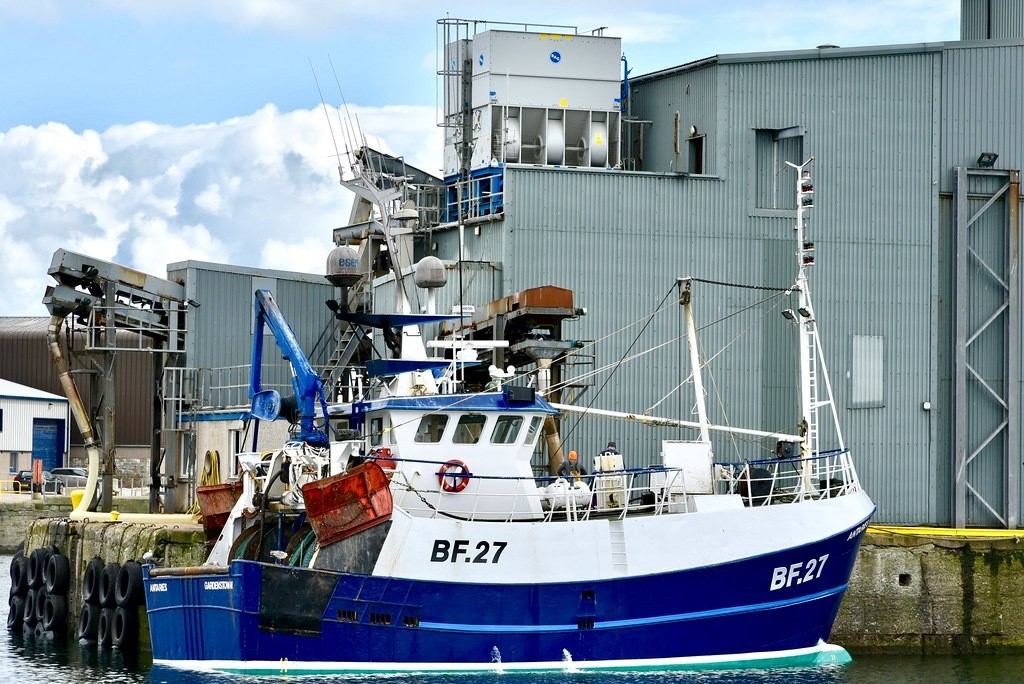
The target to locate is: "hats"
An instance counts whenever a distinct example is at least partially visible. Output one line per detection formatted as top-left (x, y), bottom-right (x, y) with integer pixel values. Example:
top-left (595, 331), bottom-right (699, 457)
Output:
top-left (569, 450), bottom-right (577, 459)
top-left (607, 442), bottom-right (616, 448)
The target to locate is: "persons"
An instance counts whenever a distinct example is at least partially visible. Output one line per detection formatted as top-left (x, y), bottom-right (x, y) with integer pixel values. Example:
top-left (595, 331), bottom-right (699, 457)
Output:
top-left (593, 442), bottom-right (625, 469)
top-left (558, 451), bottom-right (588, 482)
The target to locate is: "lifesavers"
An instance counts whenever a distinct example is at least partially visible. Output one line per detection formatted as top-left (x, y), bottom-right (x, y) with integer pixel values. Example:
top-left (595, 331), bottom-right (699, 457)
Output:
top-left (6, 545), bottom-right (143, 647)
top-left (438, 459), bottom-right (471, 492)
